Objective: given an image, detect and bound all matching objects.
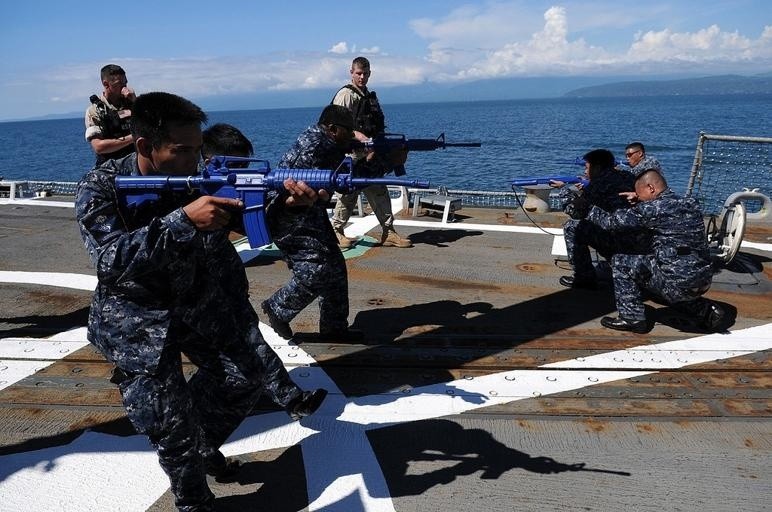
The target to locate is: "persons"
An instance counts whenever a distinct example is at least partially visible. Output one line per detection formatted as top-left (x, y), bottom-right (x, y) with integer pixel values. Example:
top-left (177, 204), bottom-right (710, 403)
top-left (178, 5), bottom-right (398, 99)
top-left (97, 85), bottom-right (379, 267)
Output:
top-left (72, 91), bottom-right (329, 512)
top-left (84, 63), bottom-right (138, 168)
top-left (189, 124), bottom-right (328, 423)
top-left (261, 105), bottom-right (407, 342)
top-left (326, 56), bottom-right (413, 249)
top-left (580, 168), bottom-right (727, 335)
top-left (549, 141), bottom-right (664, 290)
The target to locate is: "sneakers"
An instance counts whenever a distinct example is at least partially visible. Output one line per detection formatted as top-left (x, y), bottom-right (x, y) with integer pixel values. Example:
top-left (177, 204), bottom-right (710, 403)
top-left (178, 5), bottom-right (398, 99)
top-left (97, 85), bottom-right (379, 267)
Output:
top-left (600, 317), bottom-right (648, 334)
top-left (695, 302), bottom-right (726, 331)
top-left (559, 276), bottom-right (596, 289)
top-left (336, 330), bottom-right (357, 338)
top-left (260, 299), bottom-right (292, 339)
top-left (203, 449), bottom-right (226, 476)
top-left (285, 388), bottom-right (327, 420)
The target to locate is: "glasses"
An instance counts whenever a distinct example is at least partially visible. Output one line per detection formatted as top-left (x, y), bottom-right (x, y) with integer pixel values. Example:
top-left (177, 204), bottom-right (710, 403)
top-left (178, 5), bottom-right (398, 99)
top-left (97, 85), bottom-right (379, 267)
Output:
top-left (329, 123), bottom-right (353, 132)
top-left (626, 150), bottom-right (641, 157)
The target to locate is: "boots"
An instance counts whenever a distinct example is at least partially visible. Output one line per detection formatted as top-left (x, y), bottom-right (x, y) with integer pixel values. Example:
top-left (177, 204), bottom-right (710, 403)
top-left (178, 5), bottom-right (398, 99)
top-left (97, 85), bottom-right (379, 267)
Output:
top-left (381, 229), bottom-right (412, 248)
top-left (334, 231), bottom-right (353, 248)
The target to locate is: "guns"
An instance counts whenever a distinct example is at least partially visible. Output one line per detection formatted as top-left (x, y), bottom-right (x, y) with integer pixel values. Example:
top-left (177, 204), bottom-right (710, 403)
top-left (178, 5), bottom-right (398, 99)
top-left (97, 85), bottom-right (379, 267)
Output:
top-left (506, 176), bottom-right (593, 198)
top-left (115, 155), bottom-right (430, 250)
top-left (359, 132), bottom-right (483, 176)
top-left (547, 188), bottom-right (644, 210)
top-left (554, 157), bottom-right (629, 170)
